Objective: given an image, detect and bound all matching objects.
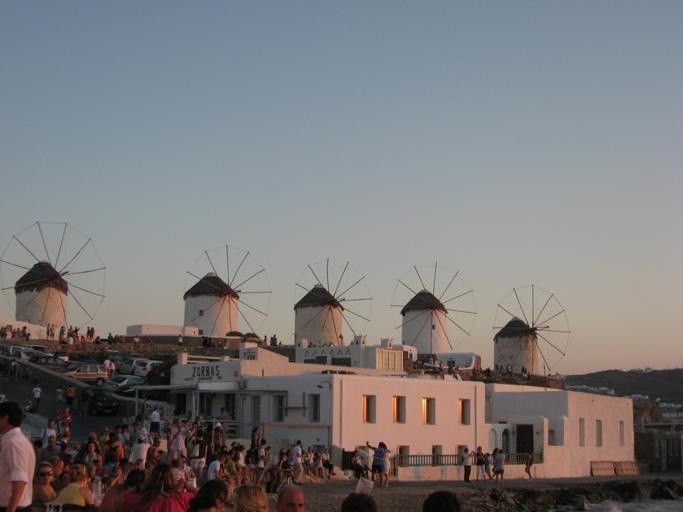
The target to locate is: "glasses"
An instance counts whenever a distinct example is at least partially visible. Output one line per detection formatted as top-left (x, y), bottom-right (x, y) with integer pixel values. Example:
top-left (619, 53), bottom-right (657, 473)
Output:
top-left (39, 434), bottom-right (227, 511)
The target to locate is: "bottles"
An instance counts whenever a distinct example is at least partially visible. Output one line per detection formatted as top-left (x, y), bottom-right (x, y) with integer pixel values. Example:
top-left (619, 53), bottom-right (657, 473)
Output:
top-left (92, 476), bottom-right (102, 497)
